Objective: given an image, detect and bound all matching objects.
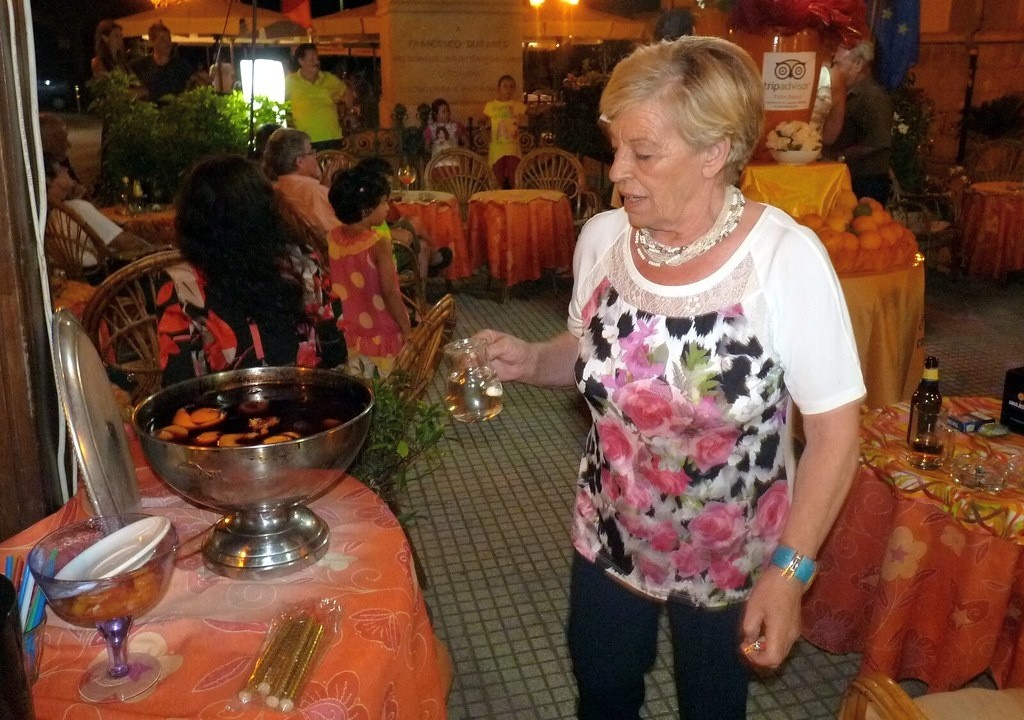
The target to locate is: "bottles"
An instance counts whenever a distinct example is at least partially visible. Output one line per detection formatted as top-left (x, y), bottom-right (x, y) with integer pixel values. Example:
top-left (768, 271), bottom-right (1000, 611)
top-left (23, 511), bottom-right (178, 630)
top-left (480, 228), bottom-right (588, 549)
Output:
top-left (118, 175), bottom-right (144, 212)
top-left (906, 356), bottom-right (943, 448)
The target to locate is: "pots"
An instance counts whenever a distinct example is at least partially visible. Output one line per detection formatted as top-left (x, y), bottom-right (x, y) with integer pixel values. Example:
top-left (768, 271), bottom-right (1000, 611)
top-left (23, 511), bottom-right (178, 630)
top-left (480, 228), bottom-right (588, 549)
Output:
top-left (131, 366), bottom-right (375, 581)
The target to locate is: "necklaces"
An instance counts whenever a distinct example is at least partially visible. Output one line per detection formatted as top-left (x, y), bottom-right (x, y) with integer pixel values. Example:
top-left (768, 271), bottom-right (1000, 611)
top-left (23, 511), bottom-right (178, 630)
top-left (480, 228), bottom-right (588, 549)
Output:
top-left (634, 182), bottom-right (744, 269)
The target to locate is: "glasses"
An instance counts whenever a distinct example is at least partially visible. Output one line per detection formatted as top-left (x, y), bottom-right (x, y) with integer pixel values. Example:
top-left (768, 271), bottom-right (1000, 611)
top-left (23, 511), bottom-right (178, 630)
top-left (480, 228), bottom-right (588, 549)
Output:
top-left (304, 150), bottom-right (317, 157)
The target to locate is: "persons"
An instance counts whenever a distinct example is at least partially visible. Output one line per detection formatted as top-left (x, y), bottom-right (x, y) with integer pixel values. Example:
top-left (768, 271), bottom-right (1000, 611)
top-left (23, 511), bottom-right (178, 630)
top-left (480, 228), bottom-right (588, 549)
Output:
top-left (132, 22), bottom-right (209, 107)
top-left (477, 72), bottom-right (566, 191)
top-left (210, 51), bottom-right (237, 97)
top-left (425, 100), bottom-right (470, 177)
top-left (808, 33), bottom-right (894, 209)
top-left (150, 153), bottom-right (343, 383)
top-left (316, 162), bottom-right (416, 393)
top-left (248, 120), bottom-right (456, 277)
top-left (92, 18), bottom-right (136, 80)
top-left (466, 33), bottom-right (865, 720)
top-left (30, 111), bottom-right (159, 312)
top-left (286, 42), bottom-right (358, 151)
top-left (430, 125), bottom-right (461, 168)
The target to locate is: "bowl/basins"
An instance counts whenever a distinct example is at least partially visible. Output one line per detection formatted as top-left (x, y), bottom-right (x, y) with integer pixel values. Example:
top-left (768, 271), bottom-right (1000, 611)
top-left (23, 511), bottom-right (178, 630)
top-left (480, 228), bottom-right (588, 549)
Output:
top-left (950, 453), bottom-right (1024, 493)
top-left (770, 149), bottom-right (818, 166)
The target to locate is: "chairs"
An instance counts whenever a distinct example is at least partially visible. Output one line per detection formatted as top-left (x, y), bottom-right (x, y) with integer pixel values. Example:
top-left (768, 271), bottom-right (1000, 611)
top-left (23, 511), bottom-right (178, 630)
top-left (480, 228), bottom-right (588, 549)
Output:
top-left (46, 141), bottom-right (1024, 587)
top-left (838, 672), bottom-right (1023, 720)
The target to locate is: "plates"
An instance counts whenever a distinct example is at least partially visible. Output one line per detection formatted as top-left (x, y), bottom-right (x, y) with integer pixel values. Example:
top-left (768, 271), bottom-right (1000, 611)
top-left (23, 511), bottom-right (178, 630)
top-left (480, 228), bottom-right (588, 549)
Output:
top-left (49, 515), bottom-right (171, 599)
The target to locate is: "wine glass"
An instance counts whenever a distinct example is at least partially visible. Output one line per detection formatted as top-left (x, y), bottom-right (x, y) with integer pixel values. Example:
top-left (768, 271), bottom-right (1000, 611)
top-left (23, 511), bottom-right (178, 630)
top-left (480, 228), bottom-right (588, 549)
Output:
top-left (27, 512), bottom-right (177, 703)
top-left (398, 165), bottom-right (416, 204)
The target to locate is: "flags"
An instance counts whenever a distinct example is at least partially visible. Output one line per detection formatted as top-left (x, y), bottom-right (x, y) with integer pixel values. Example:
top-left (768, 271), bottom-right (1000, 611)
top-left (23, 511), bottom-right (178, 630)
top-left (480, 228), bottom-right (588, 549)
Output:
top-left (867, 0), bottom-right (921, 91)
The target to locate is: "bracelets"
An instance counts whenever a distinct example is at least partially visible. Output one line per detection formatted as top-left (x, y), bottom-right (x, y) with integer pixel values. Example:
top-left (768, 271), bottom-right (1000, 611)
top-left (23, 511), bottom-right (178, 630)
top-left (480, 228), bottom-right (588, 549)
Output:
top-left (770, 544), bottom-right (822, 587)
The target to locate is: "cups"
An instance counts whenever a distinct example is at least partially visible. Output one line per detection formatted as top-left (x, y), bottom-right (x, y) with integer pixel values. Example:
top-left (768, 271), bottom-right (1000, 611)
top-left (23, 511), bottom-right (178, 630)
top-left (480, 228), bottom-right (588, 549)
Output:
top-left (443, 337), bottom-right (503, 423)
top-left (113, 188), bottom-right (165, 218)
top-left (907, 402), bottom-right (956, 471)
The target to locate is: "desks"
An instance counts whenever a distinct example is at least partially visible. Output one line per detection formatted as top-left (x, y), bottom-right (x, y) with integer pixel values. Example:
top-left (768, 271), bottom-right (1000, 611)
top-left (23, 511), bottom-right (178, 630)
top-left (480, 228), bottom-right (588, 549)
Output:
top-left (385, 191), bottom-right (473, 281)
top-left (829, 253), bottom-right (926, 408)
top-left (23, 461), bottom-right (453, 718)
top-left (789, 395), bottom-right (1023, 688)
top-left (740, 158), bottom-right (852, 219)
top-left (466, 190), bottom-right (577, 305)
top-left (961, 180), bottom-right (1024, 280)
top-left (49, 279), bottom-right (116, 363)
top-left (99, 202), bottom-right (177, 242)
